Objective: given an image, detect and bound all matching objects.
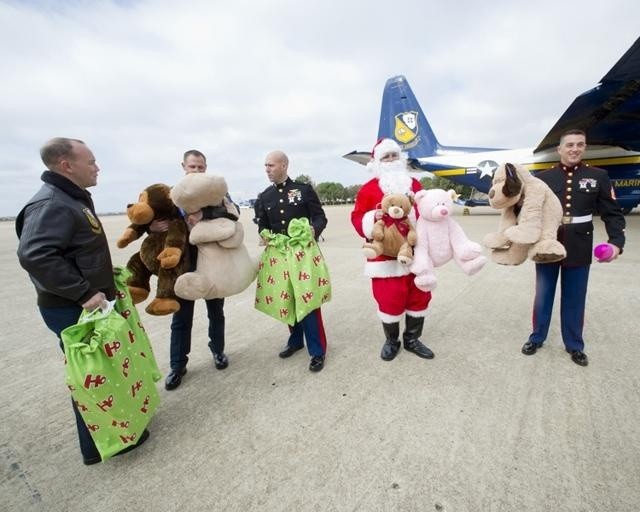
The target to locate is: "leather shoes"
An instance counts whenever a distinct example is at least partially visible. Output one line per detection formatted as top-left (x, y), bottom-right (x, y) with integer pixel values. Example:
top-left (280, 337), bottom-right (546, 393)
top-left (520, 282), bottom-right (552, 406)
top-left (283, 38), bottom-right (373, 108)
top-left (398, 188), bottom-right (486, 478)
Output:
top-left (279, 345), bottom-right (304, 358)
top-left (214, 352), bottom-right (228, 369)
top-left (310, 354), bottom-right (325, 371)
top-left (566, 344), bottom-right (588, 366)
top-left (83, 430), bottom-right (149, 466)
top-left (166, 367), bottom-right (187, 390)
top-left (522, 340), bottom-right (542, 355)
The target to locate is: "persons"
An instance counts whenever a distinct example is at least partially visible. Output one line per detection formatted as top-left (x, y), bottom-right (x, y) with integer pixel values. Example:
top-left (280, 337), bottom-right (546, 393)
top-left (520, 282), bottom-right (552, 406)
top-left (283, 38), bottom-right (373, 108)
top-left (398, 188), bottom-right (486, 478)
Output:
top-left (514, 130), bottom-right (625, 366)
top-left (349, 138), bottom-right (434, 361)
top-left (145, 149), bottom-right (241, 390)
top-left (252, 149), bottom-right (328, 371)
top-left (14, 137), bottom-right (149, 465)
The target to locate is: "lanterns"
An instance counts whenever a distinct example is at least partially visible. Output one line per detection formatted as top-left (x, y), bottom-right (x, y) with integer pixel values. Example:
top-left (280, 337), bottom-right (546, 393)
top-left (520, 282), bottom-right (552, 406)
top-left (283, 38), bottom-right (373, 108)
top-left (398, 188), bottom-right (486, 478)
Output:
top-left (485, 163), bottom-right (568, 266)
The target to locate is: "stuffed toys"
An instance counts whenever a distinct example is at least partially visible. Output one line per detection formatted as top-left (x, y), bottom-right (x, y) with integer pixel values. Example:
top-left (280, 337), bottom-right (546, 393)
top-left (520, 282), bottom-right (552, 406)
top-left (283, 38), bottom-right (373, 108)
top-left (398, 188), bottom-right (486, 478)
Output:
top-left (361, 191), bottom-right (418, 266)
top-left (169, 173), bottom-right (261, 301)
top-left (117, 183), bottom-right (191, 315)
top-left (410, 188), bottom-right (488, 292)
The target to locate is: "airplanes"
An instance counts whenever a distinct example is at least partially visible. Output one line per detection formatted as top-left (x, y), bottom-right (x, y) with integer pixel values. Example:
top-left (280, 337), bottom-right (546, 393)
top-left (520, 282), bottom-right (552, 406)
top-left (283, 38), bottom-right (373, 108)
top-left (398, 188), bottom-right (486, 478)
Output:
top-left (341, 38), bottom-right (640, 218)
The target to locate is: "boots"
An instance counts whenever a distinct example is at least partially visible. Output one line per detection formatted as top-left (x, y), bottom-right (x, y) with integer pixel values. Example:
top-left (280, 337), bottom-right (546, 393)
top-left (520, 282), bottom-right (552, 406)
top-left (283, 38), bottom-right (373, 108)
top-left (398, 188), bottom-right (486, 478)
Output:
top-left (381, 323), bottom-right (400, 360)
top-left (403, 314), bottom-right (434, 359)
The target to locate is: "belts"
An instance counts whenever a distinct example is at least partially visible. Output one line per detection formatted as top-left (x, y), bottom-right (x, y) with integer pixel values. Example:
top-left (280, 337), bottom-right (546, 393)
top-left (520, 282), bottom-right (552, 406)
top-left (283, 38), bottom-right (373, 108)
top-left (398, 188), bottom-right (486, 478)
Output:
top-left (561, 213), bottom-right (592, 224)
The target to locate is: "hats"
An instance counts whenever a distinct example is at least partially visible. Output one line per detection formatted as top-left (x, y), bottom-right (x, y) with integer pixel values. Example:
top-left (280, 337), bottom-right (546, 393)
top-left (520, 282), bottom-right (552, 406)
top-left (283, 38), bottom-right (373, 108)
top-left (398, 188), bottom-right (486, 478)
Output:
top-left (367, 138), bottom-right (401, 172)
